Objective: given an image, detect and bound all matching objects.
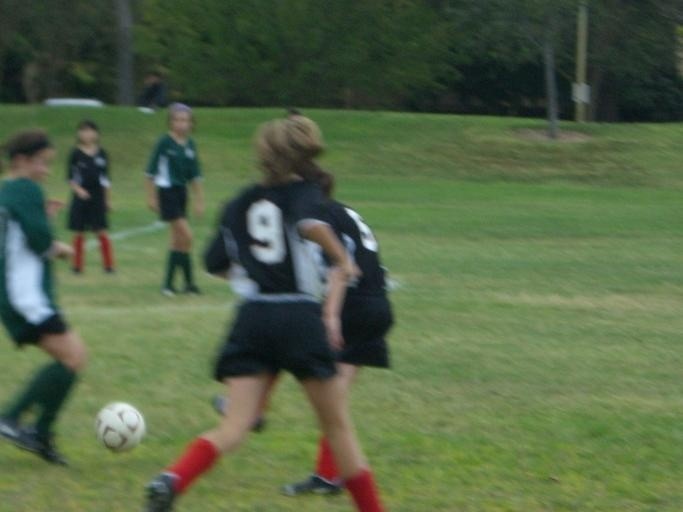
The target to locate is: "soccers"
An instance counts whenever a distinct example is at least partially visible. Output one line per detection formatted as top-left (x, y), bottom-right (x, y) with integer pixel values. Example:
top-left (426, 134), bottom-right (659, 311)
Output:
top-left (95, 403), bottom-right (145, 452)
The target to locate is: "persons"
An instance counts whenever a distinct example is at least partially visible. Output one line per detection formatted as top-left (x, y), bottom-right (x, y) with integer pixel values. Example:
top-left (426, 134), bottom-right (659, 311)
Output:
top-left (205, 159), bottom-right (395, 497)
top-left (142, 99), bottom-right (208, 300)
top-left (63, 118), bottom-right (119, 275)
top-left (136, 65), bottom-right (187, 114)
top-left (0, 126), bottom-right (88, 467)
top-left (137, 113), bottom-right (382, 512)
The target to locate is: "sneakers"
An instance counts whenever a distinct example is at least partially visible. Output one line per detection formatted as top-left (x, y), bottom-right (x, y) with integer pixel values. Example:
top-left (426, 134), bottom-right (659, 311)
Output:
top-left (14, 422), bottom-right (68, 467)
top-left (209, 394), bottom-right (266, 433)
top-left (0, 414), bottom-right (22, 443)
top-left (142, 472), bottom-right (178, 510)
top-left (70, 265), bottom-right (198, 298)
top-left (278, 472), bottom-right (344, 496)
